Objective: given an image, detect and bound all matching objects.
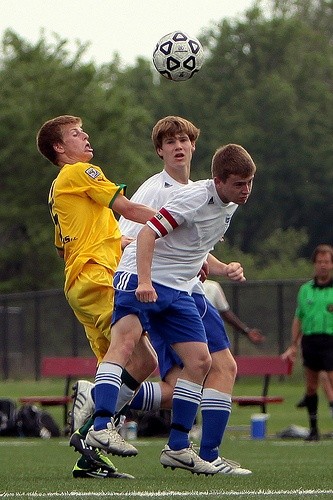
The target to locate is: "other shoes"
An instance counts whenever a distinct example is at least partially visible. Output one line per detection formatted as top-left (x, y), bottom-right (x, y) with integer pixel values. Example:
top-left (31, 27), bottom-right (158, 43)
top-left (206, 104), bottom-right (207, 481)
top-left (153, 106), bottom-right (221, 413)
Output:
top-left (305, 433), bottom-right (319, 441)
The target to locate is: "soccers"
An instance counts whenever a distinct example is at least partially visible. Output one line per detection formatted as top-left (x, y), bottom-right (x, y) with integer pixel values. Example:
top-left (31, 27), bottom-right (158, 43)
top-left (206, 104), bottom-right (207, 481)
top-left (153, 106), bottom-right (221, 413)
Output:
top-left (153, 31), bottom-right (205, 82)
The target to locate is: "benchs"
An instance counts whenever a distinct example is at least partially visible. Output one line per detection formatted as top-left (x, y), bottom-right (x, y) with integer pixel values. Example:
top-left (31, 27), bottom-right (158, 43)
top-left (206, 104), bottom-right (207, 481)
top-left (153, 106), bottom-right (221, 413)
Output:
top-left (17, 354), bottom-right (293, 433)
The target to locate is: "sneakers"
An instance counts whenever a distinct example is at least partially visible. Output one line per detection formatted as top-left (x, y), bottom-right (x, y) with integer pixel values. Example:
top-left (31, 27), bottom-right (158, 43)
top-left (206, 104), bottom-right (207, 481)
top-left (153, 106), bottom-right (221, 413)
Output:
top-left (210, 456), bottom-right (252, 475)
top-left (69, 428), bottom-right (116, 473)
top-left (85, 415), bottom-right (138, 457)
top-left (159, 440), bottom-right (219, 476)
top-left (67, 380), bottom-right (96, 437)
top-left (72, 458), bottom-right (135, 479)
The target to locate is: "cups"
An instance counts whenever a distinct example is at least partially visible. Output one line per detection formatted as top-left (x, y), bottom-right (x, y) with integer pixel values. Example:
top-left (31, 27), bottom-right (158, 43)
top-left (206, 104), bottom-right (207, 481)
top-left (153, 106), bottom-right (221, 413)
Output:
top-left (250, 413), bottom-right (264, 439)
top-left (120, 421), bottom-right (137, 440)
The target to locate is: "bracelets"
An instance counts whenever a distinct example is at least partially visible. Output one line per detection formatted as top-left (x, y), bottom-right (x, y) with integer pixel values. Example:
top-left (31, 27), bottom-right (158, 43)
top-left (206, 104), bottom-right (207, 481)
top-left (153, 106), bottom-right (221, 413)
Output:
top-left (242, 326), bottom-right (249, 335)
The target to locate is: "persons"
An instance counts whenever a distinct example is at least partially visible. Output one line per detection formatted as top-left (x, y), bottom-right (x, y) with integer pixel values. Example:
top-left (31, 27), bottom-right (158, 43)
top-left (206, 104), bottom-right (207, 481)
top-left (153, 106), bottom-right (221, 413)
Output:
top-left (187, 280), bottom-right (266, 438)
top-left (280, 329), bottom-right (333, 409)
top-left (286, 246), bottom-right (333, 442)
top-left (67, 116), bottom-right (246, 479)
top-left (84, 147), bottom-right (255, 476)
top-left (37, 116), bottom-right (158, 480)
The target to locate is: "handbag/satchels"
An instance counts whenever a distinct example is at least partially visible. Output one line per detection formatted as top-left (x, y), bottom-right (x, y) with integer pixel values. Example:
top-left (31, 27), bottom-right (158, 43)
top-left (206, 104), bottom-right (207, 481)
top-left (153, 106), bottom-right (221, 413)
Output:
top-left (0, 399), bottom-right (20, 437)
top-left (136, 409), bottom-right (197, 437)
top-left (14, 402), bottom-right (61, 438)
